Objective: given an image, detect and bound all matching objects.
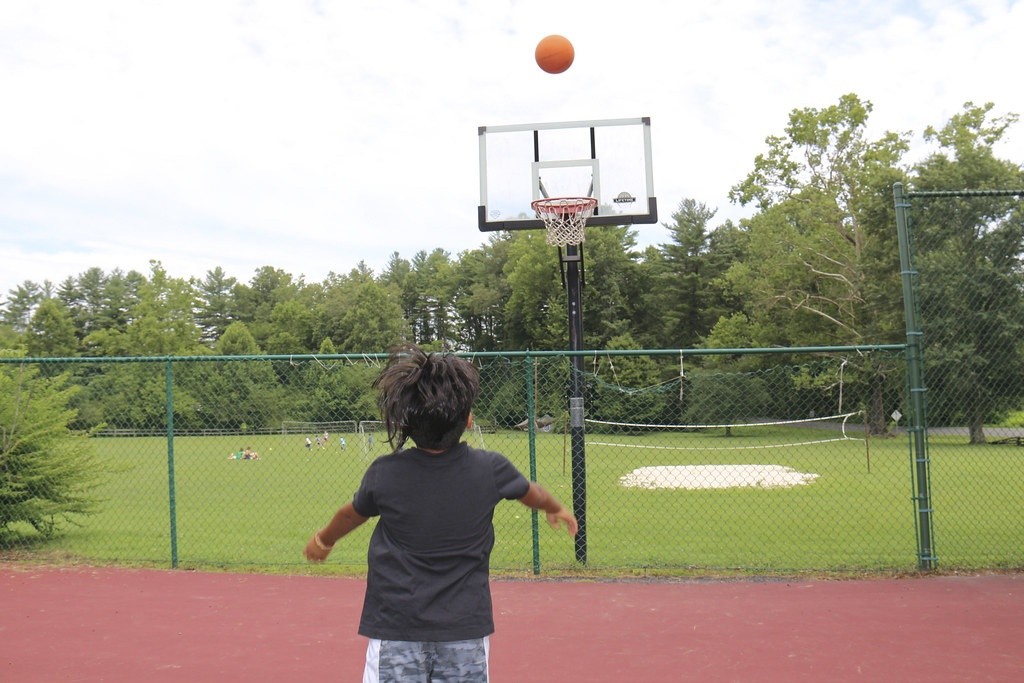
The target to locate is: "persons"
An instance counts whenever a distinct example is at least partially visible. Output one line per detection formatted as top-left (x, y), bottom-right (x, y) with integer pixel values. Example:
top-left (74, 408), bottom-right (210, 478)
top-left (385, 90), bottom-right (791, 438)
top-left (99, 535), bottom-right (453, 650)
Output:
top-left (315, 435), bottom-right (326, 451)
top-left (227, 446), bottom-right (260, 461)
top-left (306, 342), bottom-right (580, 683)
top-left (322, 430), bottom-right (332, 446)
top-left (305, 437), bottom-right (313, 452)
top-left (368, 433), bottom-right (373, 452)
top-left (340, 437), bottom-right (346, 451)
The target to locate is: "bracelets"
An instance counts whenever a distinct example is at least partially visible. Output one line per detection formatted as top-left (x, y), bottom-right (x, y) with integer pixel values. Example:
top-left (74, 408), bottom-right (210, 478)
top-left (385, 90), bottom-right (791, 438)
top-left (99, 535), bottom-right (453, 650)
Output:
top-left (314, 532), bottom-right (333, 551)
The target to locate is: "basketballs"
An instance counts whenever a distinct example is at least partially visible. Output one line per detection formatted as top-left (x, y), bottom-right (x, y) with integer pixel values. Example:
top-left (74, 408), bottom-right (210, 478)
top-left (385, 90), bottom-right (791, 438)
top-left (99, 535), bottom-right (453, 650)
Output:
top-left (535, 34), bottom-right (574, 74)
